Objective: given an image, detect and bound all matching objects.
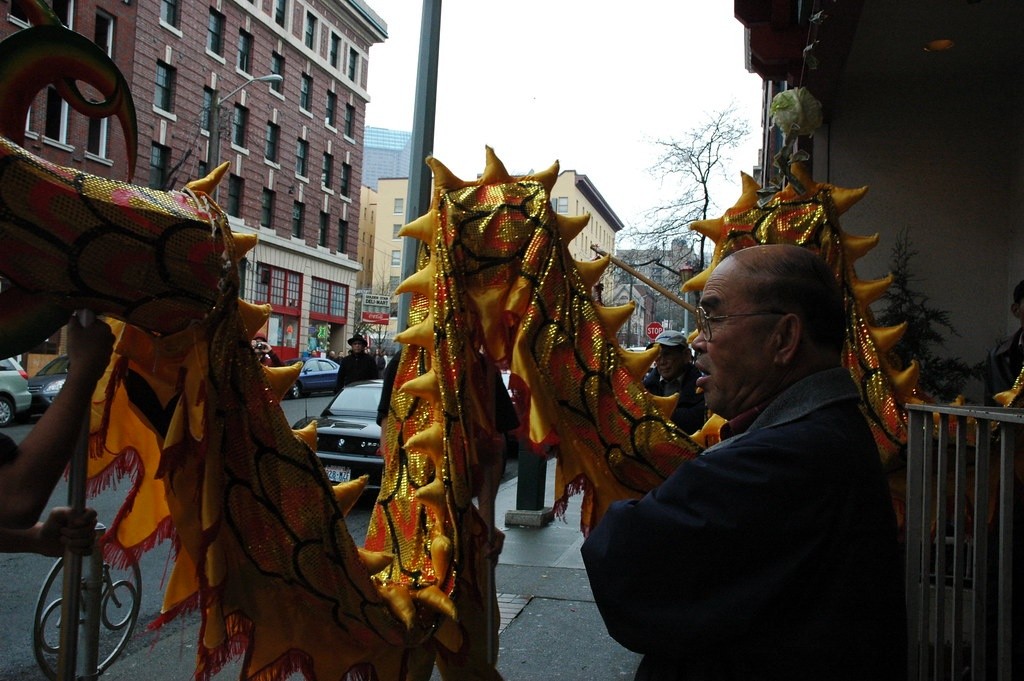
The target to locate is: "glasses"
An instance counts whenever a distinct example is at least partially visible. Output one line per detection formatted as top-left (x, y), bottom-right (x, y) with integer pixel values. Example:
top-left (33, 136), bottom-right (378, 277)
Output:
top-left (694, 306), bottom-right (787, 342)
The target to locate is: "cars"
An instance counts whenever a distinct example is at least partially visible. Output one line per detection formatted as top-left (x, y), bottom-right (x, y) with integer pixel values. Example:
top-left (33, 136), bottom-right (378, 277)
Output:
top-left (625, 346), bottom-right (657, 380)
top-left (291, 369), bottom-right (518, 491)
top-left (0, 357), bottom-right (33, 426)
top-left (282, 357), bottom-right (340, 398)
top-left (28, 353), bottom-right (69, 414)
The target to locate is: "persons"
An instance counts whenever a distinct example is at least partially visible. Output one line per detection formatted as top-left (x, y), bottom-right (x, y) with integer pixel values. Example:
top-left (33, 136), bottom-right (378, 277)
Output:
top-left (333, 333), bottom-right (380, 395)
top-left (251, 337), bottom-right (286, 367)
top-left (371, 349), bottom-right (402, 458)
top-left (578, 244), bottom-right (913, 681)
top-left (1, 315), bottom-right (117, 556)
top-left (638, 327), bottom-right (712, 438)
top-left (983, 279), bottom-right (1023, 671)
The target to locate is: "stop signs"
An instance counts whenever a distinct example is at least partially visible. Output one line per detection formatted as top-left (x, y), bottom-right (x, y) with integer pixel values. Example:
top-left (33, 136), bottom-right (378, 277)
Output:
top-left (646, 322), bottom-right (664, 341)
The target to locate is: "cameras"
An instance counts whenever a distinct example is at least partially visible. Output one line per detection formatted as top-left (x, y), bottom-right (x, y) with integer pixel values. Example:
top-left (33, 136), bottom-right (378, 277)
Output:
top-left (254, 343), bottom-right (264, 349)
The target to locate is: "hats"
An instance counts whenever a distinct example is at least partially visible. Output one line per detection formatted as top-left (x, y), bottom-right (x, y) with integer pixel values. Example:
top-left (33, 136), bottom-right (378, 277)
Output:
top-left (348, 334), bottom-right (367, 347)
top-left (646, 330), bottom-right (688, 350)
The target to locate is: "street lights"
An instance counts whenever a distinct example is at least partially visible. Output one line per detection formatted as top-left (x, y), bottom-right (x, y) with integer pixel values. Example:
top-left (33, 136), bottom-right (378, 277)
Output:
top-left (207, 73), bottom-right (284, 204)
top-left (678, 265), bottom-right (694, 339)
